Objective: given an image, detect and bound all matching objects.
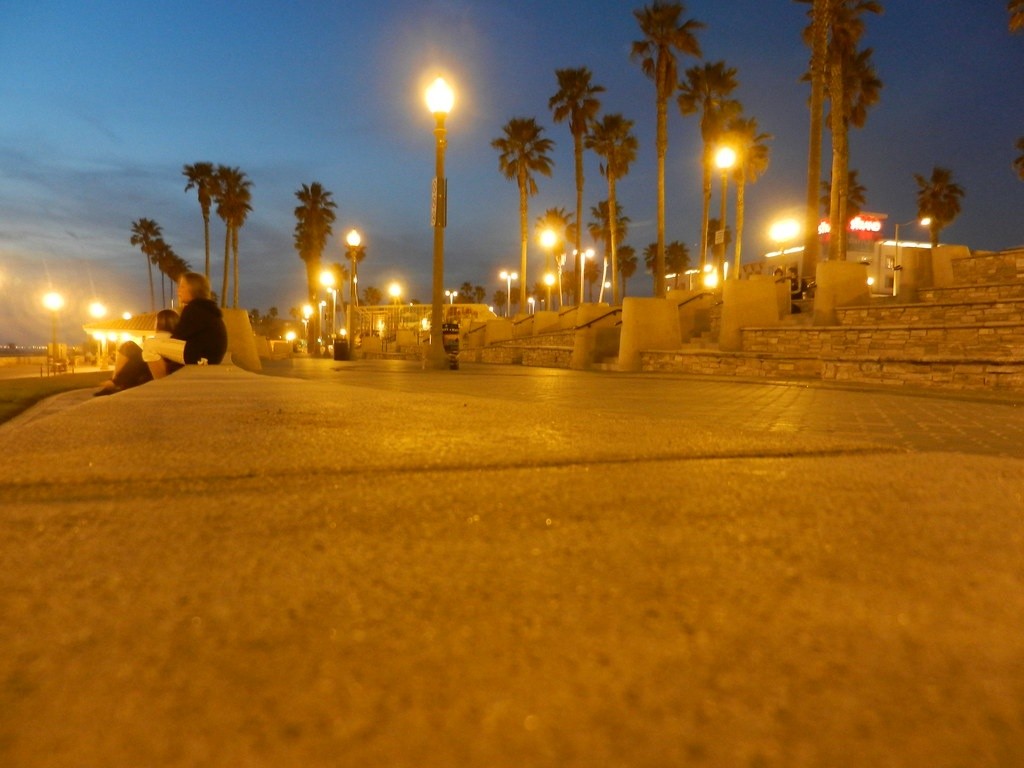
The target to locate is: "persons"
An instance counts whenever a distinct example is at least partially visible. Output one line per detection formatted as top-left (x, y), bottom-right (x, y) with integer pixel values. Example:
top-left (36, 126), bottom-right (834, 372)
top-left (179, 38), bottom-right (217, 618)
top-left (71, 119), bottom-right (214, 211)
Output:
top-left (142, 273), bottom-right (226, 382)
top-left (789, 268), bottom-right (806, 299)
top-left (94, 309), bottom-right (178, 396)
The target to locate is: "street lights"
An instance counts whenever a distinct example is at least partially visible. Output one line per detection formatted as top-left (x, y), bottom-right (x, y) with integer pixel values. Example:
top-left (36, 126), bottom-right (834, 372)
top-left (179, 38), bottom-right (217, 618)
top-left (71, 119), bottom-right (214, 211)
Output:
top-left (714, 145), bottom-right (748, 279)
top-left (422, 74), bottom-right (456, 369)
top-left (539, 226), bottom-right (564, 309)
top-left (893, 215), bottom-right (931, 295)
top-left (346, 228), bottom-right (362, 361)
top-left (43, 293), bottom-right (63, 363)
top-left (318, 300), bottom-right (328, 347)
top-left (573, 248), bottom-right (595, 304)
top-left (302, 303), bottom-right (313, 356)
top-left (326, 287), bottom-right (337, 350)
top-left (91, 300), bottom-right (108, 370)
top-left (500, 271), bottom-right (518, 319)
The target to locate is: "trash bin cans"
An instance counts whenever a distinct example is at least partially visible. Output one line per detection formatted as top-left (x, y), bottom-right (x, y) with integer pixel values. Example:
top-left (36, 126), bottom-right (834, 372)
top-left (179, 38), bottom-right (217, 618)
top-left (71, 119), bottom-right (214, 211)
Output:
top-left (333, 338), bottom-right (349, 361)
top-left (443, 323), bottom-right (459, 370)
